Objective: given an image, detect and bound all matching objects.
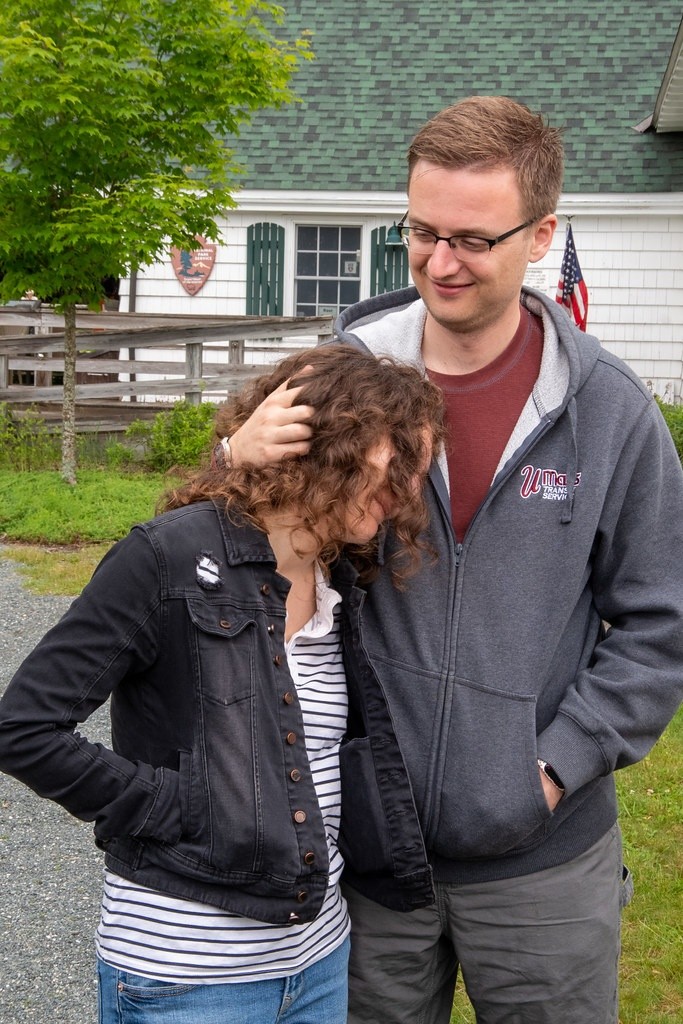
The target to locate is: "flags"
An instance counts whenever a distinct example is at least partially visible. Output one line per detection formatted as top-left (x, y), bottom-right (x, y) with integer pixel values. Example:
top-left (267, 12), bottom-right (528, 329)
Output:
top-left (556, 226), bottom-right (590, 332)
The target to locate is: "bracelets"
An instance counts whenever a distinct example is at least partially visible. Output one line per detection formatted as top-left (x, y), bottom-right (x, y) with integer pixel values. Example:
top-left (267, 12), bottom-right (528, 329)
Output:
top-left (537, 758), bottom-right (565, 793)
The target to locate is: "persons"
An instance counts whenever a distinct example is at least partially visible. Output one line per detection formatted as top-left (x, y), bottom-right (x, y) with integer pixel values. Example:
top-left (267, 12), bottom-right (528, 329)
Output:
top-left (211, 98), bottom-right (683, 1024)
top-left (0, 339), bottom-right (448, 1024)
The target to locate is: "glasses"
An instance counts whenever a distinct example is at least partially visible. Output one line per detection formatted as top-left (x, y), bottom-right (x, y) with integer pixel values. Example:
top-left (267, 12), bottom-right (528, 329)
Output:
top-left (395, 210), bottom-right (539, 262)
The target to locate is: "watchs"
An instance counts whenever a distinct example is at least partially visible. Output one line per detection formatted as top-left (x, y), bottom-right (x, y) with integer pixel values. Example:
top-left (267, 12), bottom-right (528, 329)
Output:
top-left (214, 436), bottom-right (232, 471)
